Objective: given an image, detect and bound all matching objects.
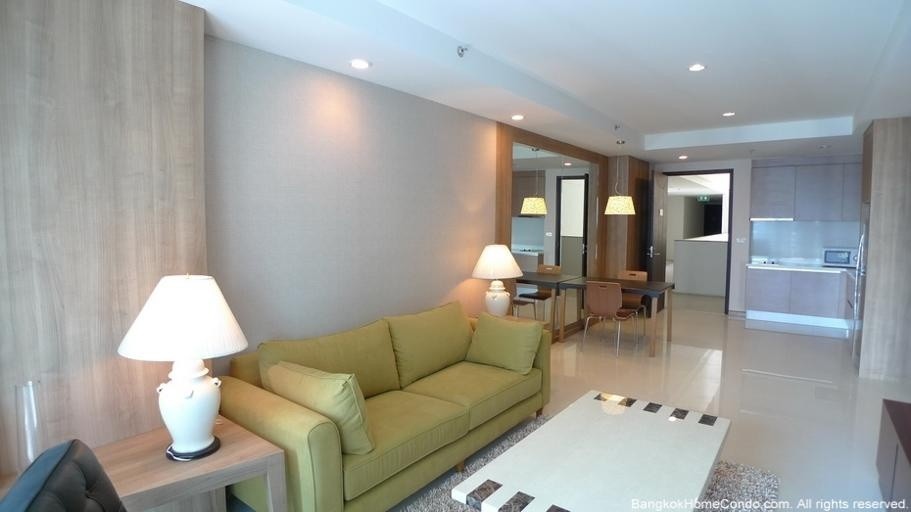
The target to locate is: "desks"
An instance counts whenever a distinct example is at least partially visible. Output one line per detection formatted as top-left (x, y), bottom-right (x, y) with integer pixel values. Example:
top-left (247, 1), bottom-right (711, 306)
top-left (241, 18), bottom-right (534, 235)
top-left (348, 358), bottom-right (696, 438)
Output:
top-left (515, 272), bottom-right (580, 337)
top-left (559, 277), bottom-right (675, 358)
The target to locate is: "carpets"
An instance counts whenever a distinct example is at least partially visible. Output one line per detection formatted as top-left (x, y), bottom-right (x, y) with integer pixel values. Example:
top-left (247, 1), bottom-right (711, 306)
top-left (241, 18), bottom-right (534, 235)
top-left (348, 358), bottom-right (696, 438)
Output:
top-left (388, 414), bottom-right (779, 512)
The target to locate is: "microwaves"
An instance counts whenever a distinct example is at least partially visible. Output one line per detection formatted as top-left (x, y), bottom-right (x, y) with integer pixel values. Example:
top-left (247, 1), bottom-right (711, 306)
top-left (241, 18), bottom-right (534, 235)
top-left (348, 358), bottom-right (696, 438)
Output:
top-left (818, 245), bottom-right (860, 269)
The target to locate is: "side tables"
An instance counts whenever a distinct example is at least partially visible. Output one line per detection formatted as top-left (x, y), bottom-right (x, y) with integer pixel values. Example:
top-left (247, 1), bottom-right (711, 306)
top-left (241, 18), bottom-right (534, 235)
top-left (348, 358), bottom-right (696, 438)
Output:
top-left (89, 413), bottom-right (288, 511)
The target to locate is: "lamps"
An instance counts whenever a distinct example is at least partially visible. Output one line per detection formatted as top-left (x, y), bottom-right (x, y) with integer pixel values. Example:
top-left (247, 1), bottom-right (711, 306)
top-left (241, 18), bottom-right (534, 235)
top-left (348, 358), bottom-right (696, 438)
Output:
top-left (117, 273), bottom-right (248, 460)
top-left (472, 245), bottom-right (523, 316)
top-left (604, 138), bottom-right (636, 215)
top-left (519, 146), bottom-right (548, 215)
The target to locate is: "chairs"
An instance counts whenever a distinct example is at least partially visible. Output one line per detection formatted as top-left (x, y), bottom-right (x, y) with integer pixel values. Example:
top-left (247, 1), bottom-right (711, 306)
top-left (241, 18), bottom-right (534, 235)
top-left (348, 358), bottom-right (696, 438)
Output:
top-left (0, 439), bottom-right (130, 511)
top-left (513, 265), bottom-right (561, 321)
top-left (583, 270), bottom-right (648, 357)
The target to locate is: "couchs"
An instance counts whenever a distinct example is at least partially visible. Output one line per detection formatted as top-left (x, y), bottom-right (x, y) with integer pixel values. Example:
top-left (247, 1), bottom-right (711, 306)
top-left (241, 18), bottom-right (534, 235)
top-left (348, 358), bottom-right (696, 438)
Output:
top-left (219, 299), bottom-right (552, 511)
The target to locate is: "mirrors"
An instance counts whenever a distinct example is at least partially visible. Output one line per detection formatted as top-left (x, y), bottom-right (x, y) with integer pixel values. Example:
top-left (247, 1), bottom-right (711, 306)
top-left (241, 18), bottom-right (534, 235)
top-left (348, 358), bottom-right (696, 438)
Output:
top-left (496, 122), bottom-right (609, 343)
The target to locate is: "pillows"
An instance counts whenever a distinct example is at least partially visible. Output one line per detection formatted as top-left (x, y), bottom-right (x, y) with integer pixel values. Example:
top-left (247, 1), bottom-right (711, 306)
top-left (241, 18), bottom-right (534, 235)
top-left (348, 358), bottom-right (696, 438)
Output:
top-left (269, 360), bottom-right (375, 455)
top-left (466, 313), bottom-right (543, 375)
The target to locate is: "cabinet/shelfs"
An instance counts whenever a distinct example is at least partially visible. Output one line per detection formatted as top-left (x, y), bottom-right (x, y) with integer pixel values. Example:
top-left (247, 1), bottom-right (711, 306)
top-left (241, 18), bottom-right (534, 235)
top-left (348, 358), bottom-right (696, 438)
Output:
top-left (512, 170), bottom-right (545, 218)
top-left (744, 270), bottom-right (844, 339)
top-left (750, 166), bottom-right (796, 221)
top-left (795, 163), bottom-right (861, 222)
top-left (845, 275), bottom-right (864, 343)
top-left (876, 398), bottom-right (910, 512)
